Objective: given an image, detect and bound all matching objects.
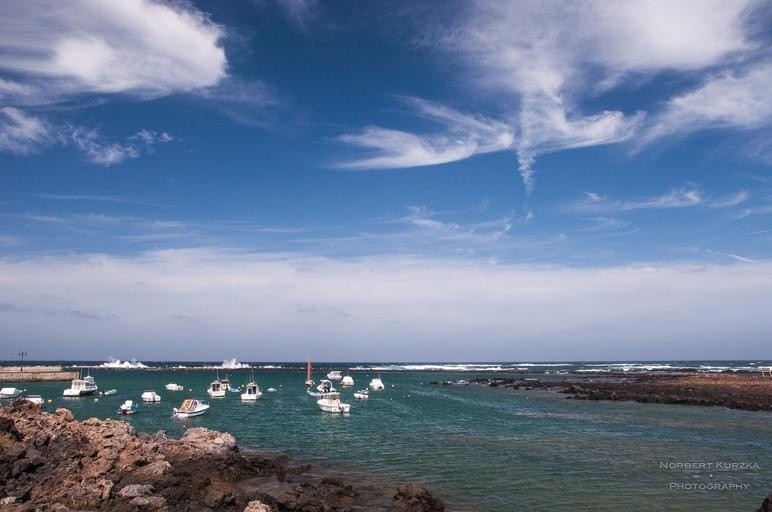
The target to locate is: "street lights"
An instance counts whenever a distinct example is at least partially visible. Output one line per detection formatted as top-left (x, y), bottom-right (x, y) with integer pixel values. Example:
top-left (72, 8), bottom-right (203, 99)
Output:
top-left (18, 351), bottom-right (27, 371)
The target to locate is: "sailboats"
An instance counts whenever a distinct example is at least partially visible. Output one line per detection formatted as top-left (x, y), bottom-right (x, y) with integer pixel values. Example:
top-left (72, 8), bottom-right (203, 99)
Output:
top-left (305, 361), bottom-right (315, 385)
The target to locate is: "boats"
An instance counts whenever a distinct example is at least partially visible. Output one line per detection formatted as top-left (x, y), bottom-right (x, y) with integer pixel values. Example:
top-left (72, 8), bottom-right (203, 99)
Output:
top-left (26, 394), bottom-right (45, 407)
top-left (0, 386), bottom-right (23, 398)
top-left (118, 400), bottom-right (139, 415)
top-left (172, 398), bottom-right (210, 418)
top-left (327, 363), bottom-right (385, 399)
top-left (165, 383), bottom-right (184, 391)
top-left (209, 367), bottom-right (230, 397)
top-left (63, 367), bottom-right (98, 397)
top-left (306, 380), bottom-right (341, 395)
top-left (142, 390), bottom-right (161, 401)
top-left (241, 368), bottom-right (263, 402)
top-left (316, 397), bottom-right (351, 412)
